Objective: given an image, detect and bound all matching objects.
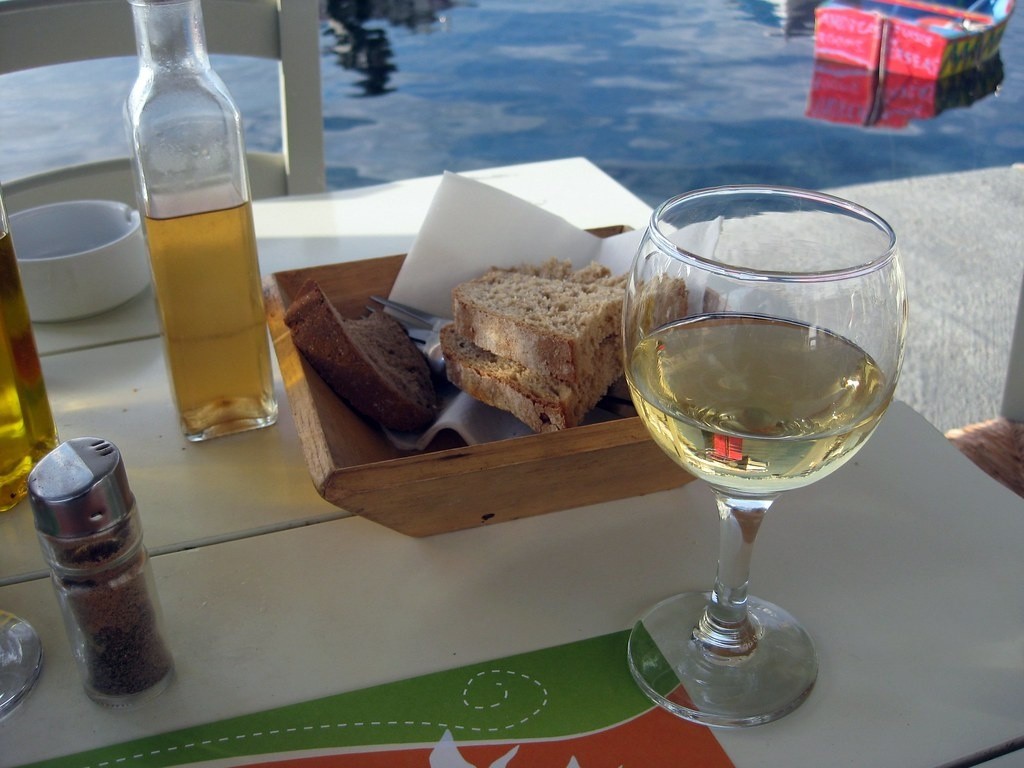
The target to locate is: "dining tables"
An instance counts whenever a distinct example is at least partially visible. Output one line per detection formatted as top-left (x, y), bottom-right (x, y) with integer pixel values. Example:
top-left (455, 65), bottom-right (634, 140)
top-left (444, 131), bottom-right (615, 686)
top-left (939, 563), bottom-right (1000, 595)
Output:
top-left (0, 155), bottom-right (1023, 768)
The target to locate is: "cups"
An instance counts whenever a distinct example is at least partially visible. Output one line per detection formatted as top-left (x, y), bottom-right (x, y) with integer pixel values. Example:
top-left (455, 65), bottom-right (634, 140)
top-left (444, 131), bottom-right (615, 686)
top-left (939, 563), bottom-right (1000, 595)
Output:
top-left (1, 200), bottom-right (61, 514)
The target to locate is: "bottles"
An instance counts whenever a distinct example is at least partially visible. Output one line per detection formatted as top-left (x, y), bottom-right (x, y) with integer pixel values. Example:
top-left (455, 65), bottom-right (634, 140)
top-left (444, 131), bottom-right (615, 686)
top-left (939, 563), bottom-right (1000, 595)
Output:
top-left (26, 436), bottom-right (176, 710)
top-left (125, 0), bottom-right (279, 443)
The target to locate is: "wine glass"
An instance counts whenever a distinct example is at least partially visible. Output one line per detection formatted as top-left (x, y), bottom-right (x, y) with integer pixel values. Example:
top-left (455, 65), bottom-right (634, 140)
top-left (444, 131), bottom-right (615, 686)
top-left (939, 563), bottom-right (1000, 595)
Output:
top-left (1, 610), bottom-right (42, 717)
top-left (621, 185), bottom-right (908, 729)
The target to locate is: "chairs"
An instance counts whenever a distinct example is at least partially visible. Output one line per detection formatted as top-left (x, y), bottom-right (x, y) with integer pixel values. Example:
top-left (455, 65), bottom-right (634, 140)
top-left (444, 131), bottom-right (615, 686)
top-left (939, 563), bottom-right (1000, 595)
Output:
top-left (1, 0), bottom-right (328, 222)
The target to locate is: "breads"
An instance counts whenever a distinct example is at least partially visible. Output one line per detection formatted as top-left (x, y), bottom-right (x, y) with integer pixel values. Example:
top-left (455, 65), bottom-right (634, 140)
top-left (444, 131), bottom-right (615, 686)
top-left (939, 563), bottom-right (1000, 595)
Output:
top-left (282, 279), bottom-right (437, 432)
top-left (440, 256), bottom-right (686, 435)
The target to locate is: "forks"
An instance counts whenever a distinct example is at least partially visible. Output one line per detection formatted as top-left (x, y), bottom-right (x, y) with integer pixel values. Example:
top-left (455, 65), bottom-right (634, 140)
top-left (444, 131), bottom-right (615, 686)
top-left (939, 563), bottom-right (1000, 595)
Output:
top-left (360, 293), bottom-right (454, 379)
